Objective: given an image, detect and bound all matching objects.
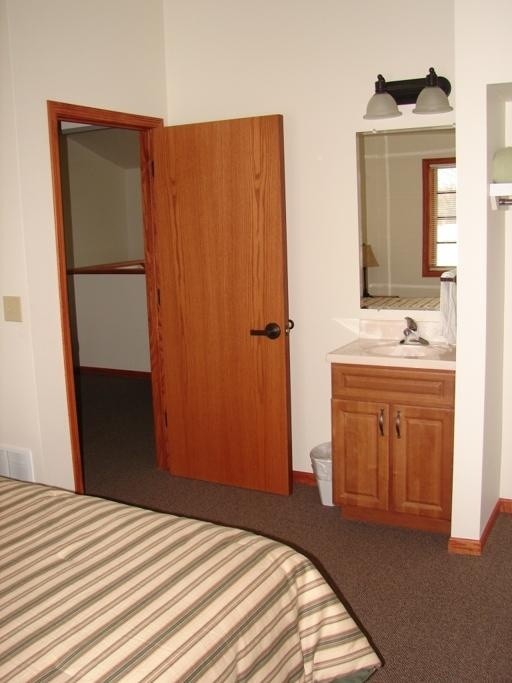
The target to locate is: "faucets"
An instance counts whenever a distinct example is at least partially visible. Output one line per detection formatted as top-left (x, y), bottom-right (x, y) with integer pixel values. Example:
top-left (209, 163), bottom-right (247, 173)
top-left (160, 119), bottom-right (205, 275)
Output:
top-left (400, 316), bottom-right (430, 346)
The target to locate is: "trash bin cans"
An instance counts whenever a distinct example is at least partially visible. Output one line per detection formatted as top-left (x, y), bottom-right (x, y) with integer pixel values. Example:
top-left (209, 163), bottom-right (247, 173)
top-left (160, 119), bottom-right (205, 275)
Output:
top-left (310, 442), bottom-right (337, 507)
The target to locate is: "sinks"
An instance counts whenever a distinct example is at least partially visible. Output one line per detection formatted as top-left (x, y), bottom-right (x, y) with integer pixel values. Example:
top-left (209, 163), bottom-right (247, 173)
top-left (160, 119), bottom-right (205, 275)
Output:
top-left (365, 344), bottom-right (438, 357)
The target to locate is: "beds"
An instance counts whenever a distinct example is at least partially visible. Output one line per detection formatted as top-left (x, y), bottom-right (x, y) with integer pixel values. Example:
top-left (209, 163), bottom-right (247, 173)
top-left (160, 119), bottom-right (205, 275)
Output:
top-left (1, 473), bottom-right (385, 683)
top-left (361, 294), bottom-right (441, 311)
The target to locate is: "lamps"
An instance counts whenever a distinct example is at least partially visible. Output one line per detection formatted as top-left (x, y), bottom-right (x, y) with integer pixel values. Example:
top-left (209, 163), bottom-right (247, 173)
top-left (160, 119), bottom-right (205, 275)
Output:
top-left (362, 66), bottom-right (455, 119)
top-left (362, 243), bottom-right (379, 296)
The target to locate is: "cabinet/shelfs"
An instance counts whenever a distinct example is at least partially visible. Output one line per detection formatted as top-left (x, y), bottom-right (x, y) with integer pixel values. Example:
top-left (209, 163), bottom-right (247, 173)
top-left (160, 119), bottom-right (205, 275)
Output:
top-left (331, 362), bottom-right (456, 534)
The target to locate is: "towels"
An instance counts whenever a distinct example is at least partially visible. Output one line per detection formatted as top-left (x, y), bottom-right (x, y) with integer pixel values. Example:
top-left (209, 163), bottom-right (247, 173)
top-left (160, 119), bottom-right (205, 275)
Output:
top-left (434, 268), bottom-right (457, 346)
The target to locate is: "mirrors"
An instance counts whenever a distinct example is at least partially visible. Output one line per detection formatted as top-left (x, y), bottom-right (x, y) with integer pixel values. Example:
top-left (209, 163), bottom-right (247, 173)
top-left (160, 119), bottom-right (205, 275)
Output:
top-left (354, 124), bottom-right (458, 312)
